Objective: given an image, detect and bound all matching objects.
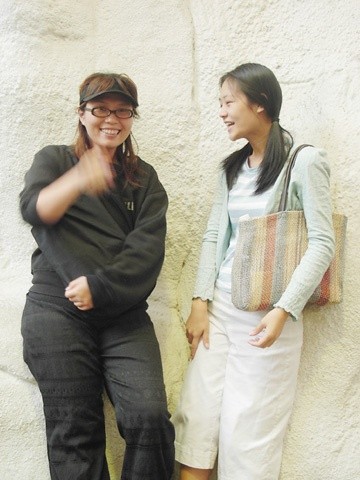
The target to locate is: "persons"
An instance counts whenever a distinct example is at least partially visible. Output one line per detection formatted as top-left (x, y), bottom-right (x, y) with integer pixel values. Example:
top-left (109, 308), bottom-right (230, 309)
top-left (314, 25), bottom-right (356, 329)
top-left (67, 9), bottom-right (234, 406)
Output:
top-left (170, 63), bottom-right (337, 480)
top-left (19, 72), bottom-right (177, 480)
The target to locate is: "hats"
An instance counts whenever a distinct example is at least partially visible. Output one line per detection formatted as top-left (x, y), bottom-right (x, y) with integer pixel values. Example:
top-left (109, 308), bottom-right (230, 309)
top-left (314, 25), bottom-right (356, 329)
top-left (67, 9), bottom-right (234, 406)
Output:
top-left (79, 89), bottom-right (139, 108)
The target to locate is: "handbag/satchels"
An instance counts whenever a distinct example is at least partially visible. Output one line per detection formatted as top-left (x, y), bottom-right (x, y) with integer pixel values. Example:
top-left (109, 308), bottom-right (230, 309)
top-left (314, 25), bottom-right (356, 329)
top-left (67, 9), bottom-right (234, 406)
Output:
top-left (231, 145), bottom-right (349, 312)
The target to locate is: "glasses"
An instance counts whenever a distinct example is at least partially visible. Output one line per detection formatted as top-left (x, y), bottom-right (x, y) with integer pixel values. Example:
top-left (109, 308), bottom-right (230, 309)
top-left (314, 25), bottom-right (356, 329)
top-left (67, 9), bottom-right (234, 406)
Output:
top-left (80, 105), bottom-right (136, 119)
